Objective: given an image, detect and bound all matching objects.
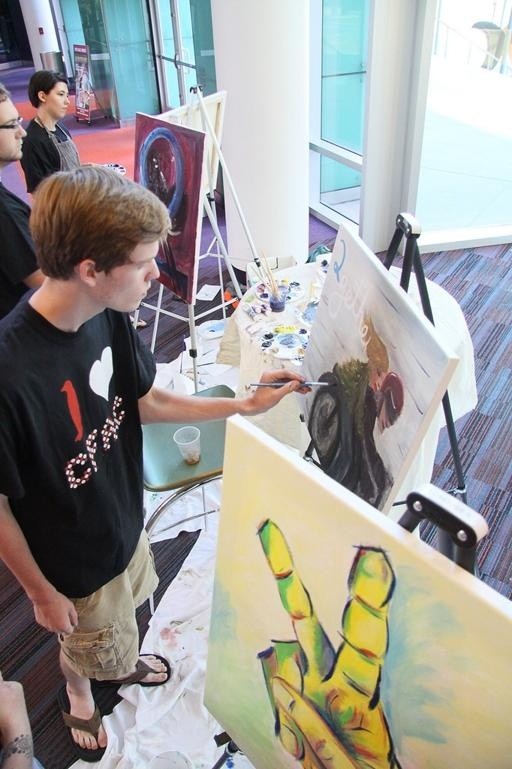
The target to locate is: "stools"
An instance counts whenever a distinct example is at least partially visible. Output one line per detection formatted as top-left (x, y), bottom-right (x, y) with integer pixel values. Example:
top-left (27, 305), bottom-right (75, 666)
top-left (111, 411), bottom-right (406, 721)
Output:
top-left (143, 384), bottom-right (235, 538)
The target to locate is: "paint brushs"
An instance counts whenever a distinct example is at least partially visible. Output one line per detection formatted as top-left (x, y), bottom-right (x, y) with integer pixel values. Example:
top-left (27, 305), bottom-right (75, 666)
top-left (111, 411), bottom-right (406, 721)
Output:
top-left (250, 381), bottom-right (336, 386)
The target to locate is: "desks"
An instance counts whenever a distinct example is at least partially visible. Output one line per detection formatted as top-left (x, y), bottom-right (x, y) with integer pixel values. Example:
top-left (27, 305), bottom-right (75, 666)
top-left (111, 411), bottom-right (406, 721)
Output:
top-left (212, 262), bottom-right (477, 454)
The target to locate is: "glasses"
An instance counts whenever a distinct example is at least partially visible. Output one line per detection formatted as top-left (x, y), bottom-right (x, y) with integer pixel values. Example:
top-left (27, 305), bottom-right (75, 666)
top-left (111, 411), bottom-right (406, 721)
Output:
top-left (0, 116), bottom-right (23, 129)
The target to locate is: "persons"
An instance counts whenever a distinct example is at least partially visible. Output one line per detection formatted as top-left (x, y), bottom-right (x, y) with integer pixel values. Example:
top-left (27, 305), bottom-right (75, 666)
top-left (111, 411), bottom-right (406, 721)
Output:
top-left (0, 79), bottom-right (47, 322)
top-left (0, 669), bottom-right (46, 768)
top-left (20, 70), bottom-right (80, 207)
top-left (0, 165), bottom-right (313, 763)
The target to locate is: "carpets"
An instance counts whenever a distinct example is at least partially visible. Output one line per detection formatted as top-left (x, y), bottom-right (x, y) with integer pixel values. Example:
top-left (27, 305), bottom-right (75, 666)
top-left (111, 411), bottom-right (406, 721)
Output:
top-left (14, 122), bottom-right (135, 204)
top-left (13, 93), bottom-right (97, 122)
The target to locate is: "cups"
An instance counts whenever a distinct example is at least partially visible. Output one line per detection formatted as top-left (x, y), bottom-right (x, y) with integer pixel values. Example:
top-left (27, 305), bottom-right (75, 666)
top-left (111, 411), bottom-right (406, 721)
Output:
top-left (173, 426), bottom-right (201, 465)
top-left (266, 283), bottom-right (288, 314)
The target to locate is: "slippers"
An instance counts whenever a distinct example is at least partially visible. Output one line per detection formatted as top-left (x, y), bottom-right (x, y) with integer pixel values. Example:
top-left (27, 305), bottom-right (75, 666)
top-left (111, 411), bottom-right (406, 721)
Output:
top-left (95, 654), bottom-right (171, 687)
top-left (56, 685), bottom-right (107, 762)
